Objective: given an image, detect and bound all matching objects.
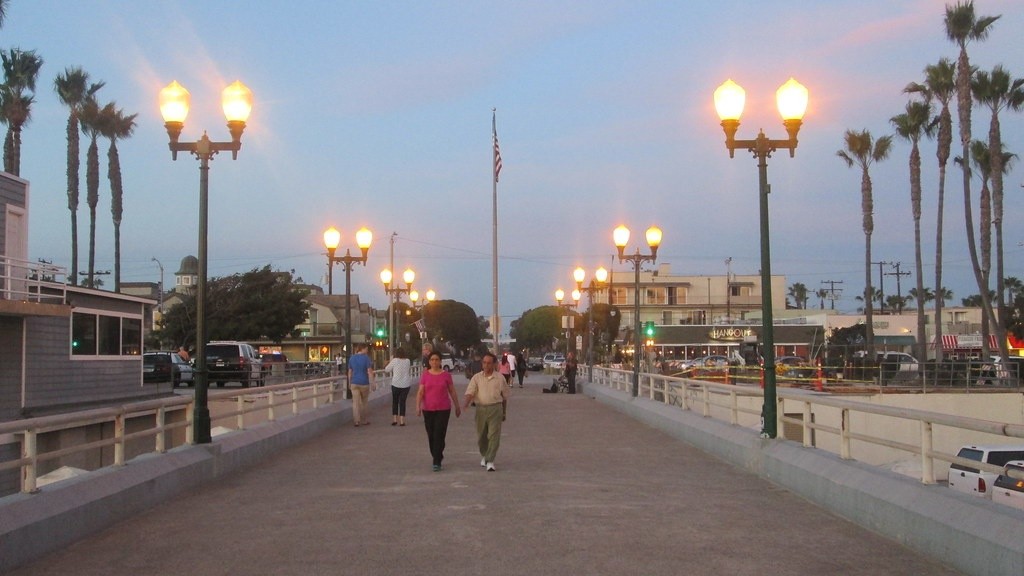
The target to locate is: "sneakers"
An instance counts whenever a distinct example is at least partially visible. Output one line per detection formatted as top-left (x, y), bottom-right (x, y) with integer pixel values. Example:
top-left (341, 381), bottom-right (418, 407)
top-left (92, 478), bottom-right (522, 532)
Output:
top-left (480, 456), bottom-right (487, 467)
top-left (486, 461), bottom-right (496, 471)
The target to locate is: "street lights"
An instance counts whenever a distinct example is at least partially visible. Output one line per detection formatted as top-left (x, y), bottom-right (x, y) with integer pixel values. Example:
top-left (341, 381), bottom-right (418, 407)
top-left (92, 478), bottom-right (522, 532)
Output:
top-left (156, 78), bottom-right (255, 443)
top-left (380, 268), bottom-right (415, 348)
top-left (573, 264), bottom-right (609, 382)
top-left (409, 288), bottom-right (436, 363)
top-left (322, 224), bottom-right (376, 400)
top-left (152, 256), bottom-right (164, 352)
top-left (612, 224), bottom-right (663, 396)
top-left (713, 76), bottom-right (813, 437)
top-left (553, 288), bottom-right (582, 356)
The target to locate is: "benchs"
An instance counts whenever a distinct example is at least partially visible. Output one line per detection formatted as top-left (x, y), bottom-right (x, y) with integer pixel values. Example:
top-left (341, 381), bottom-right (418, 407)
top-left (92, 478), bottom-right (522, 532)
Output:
top-left (553, 374), bottom-right (570, 393)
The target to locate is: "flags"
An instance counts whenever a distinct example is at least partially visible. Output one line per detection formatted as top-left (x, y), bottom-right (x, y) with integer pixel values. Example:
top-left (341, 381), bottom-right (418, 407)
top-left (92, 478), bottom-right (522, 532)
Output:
top-left (490, 123), bottom-right (504, 182)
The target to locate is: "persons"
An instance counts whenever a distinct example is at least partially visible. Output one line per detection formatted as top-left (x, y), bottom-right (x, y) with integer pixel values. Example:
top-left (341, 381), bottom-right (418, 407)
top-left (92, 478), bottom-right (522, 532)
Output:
top-left (421, 343), bottom-right (443, 371)
top-left (463, 354), bottom-right (510, 472)
top-left (416, 351), bottom-right (460, 471)
top-left (347, 343), bottom-right (376, 427)
top-left (178, 347), bottom-right (189, 360)
top-left (564, 351), bottom-right (577, 394)
top-left (468, 354), bottom-right (483, 406)
top-left (335, 353), bottom-right (344, 375)
top-left (384, 347), bottom-right (411, 426)
top-left (497, 352), bottom-right (527, 388)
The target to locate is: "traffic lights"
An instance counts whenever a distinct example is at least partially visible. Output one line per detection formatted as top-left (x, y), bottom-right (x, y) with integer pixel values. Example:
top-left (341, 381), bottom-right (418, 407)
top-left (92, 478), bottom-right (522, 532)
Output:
top-left (376, 323), bottom-right (385, 340)
top-left (645, 320), bottom-right (655, 349)
top-left (319, 345), bottom-right (330, 355)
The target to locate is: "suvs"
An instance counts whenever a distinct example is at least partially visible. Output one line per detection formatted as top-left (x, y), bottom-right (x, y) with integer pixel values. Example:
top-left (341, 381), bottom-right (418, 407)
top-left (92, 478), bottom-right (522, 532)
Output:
top-left (143, 348), bottom-right (193, 390)
top-left (945, 442), bottom-right (1024, 499)
top-left (990, 458), bottom-right (1024, 512)
top-left (190, 341), bottom-right (266, 388)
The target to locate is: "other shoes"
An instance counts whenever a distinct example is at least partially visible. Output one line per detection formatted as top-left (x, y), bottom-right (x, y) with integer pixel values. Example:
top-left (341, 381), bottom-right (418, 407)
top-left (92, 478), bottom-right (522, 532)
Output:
top-left (432, 464), bottom-right (441, 472)
top-left (354, 422), bottom-right (359, 427)
top-left (360, 422), bottom-right (370, 426)
top-left (391, 422), bottom-right (397, 426)
top-left (400, 423), bottom-right (405, 426)
top-left (519, 385), bottom-right (524, 388)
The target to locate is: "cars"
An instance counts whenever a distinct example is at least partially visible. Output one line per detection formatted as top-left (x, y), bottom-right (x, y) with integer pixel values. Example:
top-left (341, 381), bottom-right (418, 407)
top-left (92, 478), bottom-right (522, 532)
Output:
top-left (412, 353), bottom-right (470, 373)
top-left (658, 351), bottom-right (1024, 386)
top-left (522, 348), bottom-right (566, 371)
top-left (260, 352), bottom-right (290, 375)
